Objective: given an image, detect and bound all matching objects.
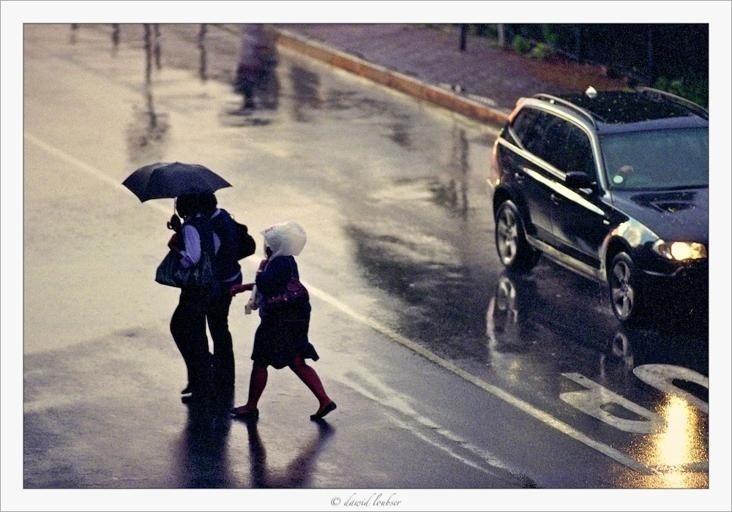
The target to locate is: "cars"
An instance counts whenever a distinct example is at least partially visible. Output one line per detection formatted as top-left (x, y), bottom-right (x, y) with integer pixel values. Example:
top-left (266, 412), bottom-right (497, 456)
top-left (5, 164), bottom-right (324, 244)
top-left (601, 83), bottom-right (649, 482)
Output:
top-left (487, 86), bottom-right (709, 326)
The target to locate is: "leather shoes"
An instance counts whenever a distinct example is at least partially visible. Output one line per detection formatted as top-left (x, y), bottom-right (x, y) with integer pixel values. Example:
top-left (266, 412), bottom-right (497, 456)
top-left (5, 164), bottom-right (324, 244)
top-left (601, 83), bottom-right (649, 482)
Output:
top-left (309, 401), bottom-right (337, 423)
top-left (229, 406), bottom-right (259, 419)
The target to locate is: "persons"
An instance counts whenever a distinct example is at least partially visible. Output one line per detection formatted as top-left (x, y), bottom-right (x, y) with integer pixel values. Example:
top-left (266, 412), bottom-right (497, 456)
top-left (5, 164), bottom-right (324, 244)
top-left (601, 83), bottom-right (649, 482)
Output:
top-left (181, 409), bottom-right (238, 489)
top-left (165, 186), bottom-right (224, 411)
top-left (227, 220), bottom-right (337, 421)
top-left (197, 192), bottom-right (249, 399)
top-left (227, 23), bottom-right (284, 122)
top-left (231, 411), bottom-right (338, 487)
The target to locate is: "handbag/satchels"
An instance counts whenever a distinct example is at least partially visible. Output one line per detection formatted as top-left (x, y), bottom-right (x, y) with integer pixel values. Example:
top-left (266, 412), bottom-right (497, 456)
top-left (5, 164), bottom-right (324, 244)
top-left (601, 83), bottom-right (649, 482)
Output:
top-left (217, 213), bottom-right (257, 267)
top-left (153, 220), bottom-right (212, 297)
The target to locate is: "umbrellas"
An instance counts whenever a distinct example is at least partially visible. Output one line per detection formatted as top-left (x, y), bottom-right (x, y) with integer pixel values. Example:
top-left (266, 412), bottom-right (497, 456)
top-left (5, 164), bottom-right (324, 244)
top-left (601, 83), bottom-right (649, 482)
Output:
top-left (119, 161), bottom-right (233, 231)
